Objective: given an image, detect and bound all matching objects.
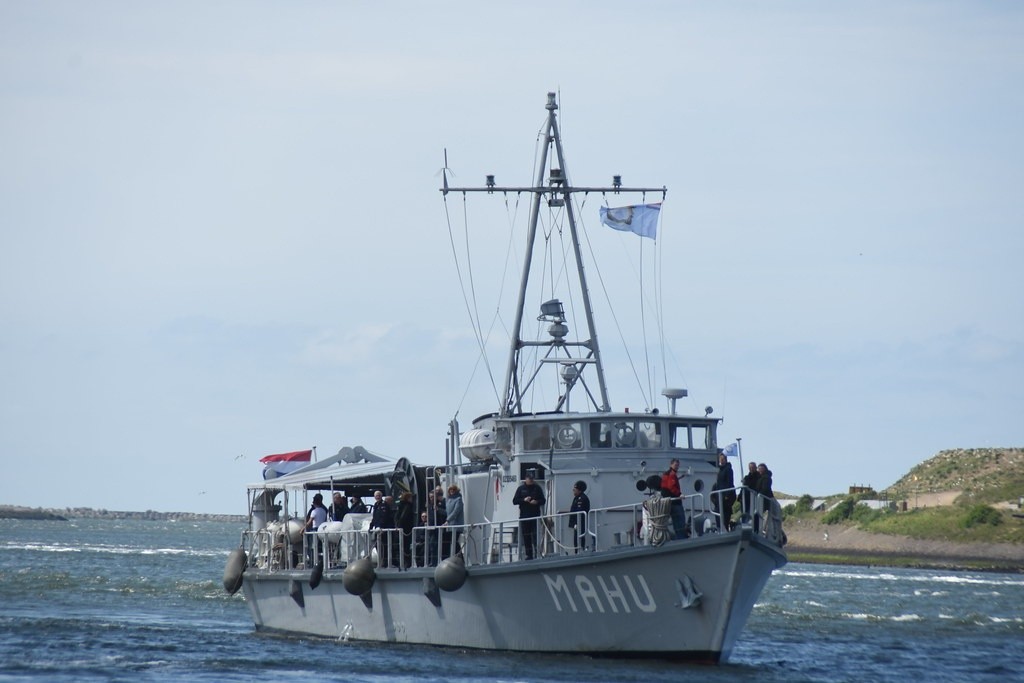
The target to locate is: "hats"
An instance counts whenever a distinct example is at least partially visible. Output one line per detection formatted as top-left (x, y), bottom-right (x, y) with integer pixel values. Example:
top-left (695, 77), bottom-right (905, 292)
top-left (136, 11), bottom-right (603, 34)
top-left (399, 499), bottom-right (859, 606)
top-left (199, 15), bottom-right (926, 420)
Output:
top-left (526, 469), bottom-right (536, 479)
top-left (574, 481), bottom-right (587, 492)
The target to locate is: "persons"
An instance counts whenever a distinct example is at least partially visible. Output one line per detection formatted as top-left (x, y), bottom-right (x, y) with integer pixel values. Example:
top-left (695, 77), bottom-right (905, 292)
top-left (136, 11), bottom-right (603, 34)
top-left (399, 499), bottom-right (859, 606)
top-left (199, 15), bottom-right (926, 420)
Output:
top-left (568, 481), bottom-right (590, 554)
top-left (713, 454), bottom-right (736, 531)
top-left (300, 485), bottom-right (464, 565)
top-left (737, 462), bottom-right (773, 533)
top-left (530, 427), bottom-right (559, 449)
top-left (513, 472), bottom-right (546, 560)
top-left (661, 460), bottom-right (685, 538)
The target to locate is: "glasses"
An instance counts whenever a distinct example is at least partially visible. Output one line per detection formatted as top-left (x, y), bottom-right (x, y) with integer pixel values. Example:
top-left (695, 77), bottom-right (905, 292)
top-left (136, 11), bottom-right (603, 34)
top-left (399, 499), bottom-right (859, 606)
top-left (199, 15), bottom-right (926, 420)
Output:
top-left (449, 488), bottom-right (456, 490)
top-left (421, 516), bottom-right (427, 518)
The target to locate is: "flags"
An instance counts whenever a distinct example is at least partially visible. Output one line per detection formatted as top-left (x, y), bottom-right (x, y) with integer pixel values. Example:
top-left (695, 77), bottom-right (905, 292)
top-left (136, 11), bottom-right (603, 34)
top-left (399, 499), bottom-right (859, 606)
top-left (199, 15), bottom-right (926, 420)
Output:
top-left (260, 450), bottom-right (311, 464)
top-left (723, 443), bottom-right (738, 456)
top-left (600, 203), bottom-right (662, 239)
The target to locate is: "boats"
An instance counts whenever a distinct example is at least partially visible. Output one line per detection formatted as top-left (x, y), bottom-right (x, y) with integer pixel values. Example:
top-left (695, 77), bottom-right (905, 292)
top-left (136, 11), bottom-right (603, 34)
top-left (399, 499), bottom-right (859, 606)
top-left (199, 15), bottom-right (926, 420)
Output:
top-left (223, 85), bottom-right (786, 665)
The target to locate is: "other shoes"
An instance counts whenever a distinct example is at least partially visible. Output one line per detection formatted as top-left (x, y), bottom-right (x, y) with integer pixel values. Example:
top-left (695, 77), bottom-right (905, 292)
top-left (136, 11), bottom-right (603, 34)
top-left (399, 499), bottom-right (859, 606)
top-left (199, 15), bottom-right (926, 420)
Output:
top-left (524, 555), bottom-right (533, 560)
